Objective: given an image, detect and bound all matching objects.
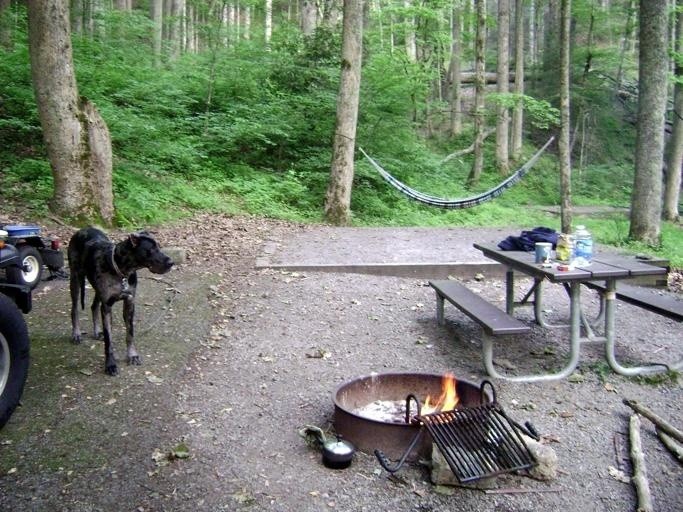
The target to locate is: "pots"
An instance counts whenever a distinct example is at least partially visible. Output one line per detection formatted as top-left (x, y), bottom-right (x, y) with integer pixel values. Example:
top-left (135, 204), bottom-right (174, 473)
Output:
top-left (308, 430), bottom-right (356, 469)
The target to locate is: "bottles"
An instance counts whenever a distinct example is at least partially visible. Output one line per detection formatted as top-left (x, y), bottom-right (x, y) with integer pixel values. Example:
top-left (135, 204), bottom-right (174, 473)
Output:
top-left (570, 225), bottom-right (593, 267)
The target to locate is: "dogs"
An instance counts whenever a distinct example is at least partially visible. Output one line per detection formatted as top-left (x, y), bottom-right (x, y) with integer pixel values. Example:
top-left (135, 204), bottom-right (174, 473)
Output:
top-left (65, 222), bottom-right (176, 376)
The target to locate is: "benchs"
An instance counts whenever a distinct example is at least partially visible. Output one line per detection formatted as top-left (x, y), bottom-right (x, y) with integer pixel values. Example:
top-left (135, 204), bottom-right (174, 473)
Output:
top-left (581, 280), bottom-right (682, 373)
top-left (426, 277), bottom-right (531, 380)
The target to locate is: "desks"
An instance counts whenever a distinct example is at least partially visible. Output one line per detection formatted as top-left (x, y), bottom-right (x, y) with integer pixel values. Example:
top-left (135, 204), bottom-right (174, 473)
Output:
top-left (472, 240), bottom-right (667, 383)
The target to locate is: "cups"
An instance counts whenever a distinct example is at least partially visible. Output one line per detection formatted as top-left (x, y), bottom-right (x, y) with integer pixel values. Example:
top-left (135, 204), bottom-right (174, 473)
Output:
top-left (533, 242), bottom-right (553, 265)
